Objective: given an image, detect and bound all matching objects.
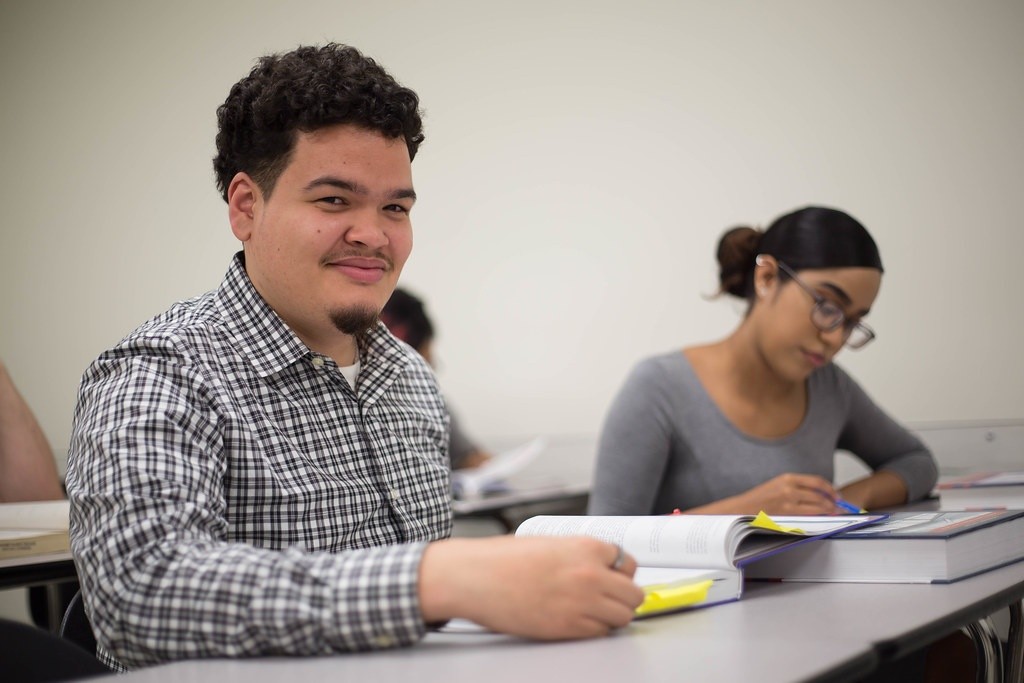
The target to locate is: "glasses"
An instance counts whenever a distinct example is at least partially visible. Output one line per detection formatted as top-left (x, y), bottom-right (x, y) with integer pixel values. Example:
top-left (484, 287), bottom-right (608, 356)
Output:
top-left (777, 261), bottom-right (876, 350)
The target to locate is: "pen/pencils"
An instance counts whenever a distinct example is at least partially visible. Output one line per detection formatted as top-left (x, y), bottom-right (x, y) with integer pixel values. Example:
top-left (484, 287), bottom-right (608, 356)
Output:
top-left (837, 501), bottom-right (867, 514)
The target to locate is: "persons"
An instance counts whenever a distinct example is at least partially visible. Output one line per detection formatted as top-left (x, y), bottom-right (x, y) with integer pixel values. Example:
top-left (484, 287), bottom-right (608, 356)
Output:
top-left (0, 359), bottom-right (65, 502)
top-left (64, 39), bottom-right (644, 675)
top-left (586, 206), bottom-right (939, 516)
top-left (378, 288), bottom-right (494, 470)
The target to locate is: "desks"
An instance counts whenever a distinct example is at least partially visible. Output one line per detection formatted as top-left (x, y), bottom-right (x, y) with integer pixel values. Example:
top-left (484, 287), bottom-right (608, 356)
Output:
top-left (70, 557), bottom-right (1024, 683)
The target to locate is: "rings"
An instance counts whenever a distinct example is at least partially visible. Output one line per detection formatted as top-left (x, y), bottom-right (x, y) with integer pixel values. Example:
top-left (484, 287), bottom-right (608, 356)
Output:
top-left (610, 544), bottom-right (624, 568)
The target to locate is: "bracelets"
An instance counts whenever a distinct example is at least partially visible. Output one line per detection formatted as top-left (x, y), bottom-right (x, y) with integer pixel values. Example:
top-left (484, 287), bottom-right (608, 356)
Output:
top-left (666, 508), bottom-right (682, 516)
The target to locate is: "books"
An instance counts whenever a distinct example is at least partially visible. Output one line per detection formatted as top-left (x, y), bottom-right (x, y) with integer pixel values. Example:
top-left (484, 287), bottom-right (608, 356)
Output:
top-left (1, 499), bottom-right (70, 559)
top-left (937, 470), bottom-right (1024, 512)
top-left (452, 443), bottom-right (539, 498)
top-left (440, 510), bottom-right (1023, 628)
